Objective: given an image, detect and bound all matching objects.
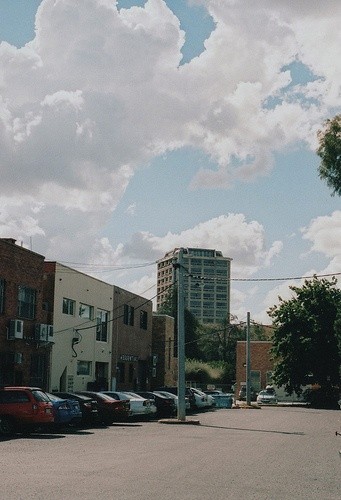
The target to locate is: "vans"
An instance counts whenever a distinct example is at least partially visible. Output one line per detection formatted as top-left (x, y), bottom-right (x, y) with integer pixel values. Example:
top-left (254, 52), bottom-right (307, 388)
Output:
top-left (0, 386), bottom-right (56, 437)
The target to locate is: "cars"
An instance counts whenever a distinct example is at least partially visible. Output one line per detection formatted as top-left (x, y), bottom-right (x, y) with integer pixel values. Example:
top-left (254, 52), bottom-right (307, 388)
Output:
top-left (238, 386), bottom-right (256, 401)
top-left (255, 389), bottom-right (278, 406)
top-left (45, 386), bottom-right (236, 432)
